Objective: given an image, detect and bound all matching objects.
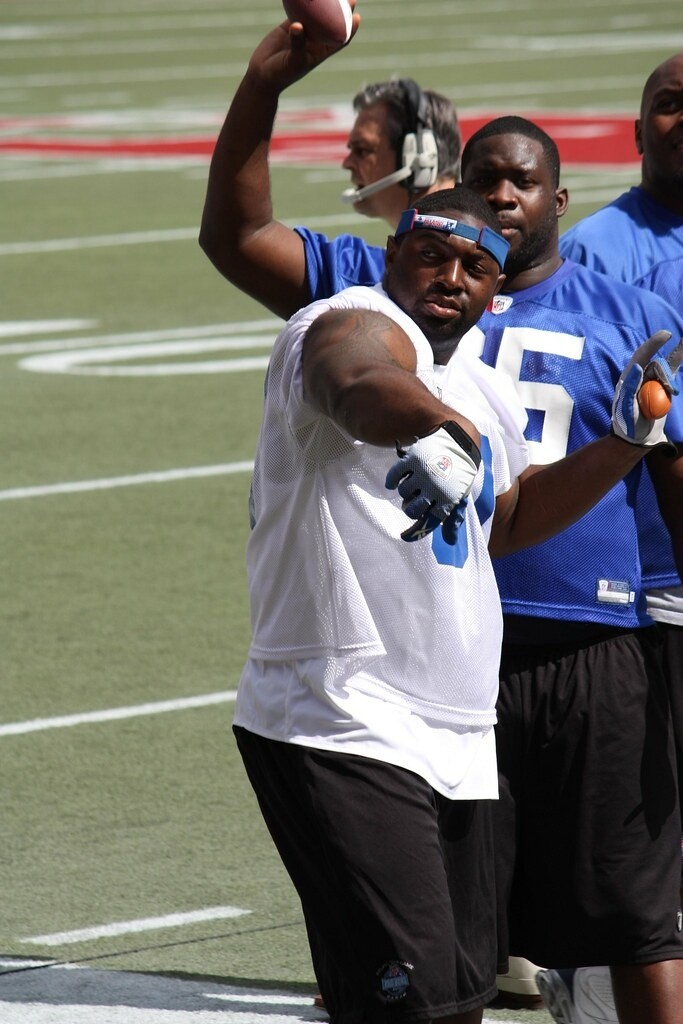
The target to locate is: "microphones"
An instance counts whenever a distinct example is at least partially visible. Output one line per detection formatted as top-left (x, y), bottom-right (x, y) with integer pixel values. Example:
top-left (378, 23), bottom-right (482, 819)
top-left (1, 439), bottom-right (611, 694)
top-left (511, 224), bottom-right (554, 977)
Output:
top-left (342, 166), bottom-right (411, 205)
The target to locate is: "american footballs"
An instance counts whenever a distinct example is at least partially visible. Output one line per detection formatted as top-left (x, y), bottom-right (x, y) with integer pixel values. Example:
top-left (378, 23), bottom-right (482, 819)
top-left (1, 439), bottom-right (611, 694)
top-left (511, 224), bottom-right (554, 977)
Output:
top-left (282, 0), bottom-right (354, 45)
top-left (638, 381), bottom-right (671, 420)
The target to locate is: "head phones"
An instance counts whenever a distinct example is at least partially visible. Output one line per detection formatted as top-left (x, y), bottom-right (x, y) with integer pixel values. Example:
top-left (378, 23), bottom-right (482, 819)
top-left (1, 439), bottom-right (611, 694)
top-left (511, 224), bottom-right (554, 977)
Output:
top-left (396, 79), bottom-right (438, 191)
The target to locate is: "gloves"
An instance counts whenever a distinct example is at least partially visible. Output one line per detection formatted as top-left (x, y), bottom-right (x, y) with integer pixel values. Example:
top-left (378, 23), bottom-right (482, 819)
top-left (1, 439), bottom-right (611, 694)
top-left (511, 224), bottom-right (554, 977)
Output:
top-left (386, 417), bottom-right (481, 545)
top-left (613, 330), bottom-right (683, 456)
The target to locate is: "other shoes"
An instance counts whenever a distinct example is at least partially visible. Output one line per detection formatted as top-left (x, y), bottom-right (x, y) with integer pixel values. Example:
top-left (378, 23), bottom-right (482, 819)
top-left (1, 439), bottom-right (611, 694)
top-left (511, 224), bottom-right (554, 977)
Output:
top-left (535, 966), bottom-right (584, 1024)
top-left (484, 956), bottom-right (544, 1009)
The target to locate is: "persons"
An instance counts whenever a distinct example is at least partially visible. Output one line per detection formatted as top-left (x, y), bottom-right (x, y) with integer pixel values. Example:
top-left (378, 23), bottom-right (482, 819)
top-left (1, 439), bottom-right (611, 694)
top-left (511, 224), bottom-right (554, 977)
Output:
top-left (231, 189), bottom-right (683, 1024)
top-left (197, 0), bottom-right (683, 1024)
